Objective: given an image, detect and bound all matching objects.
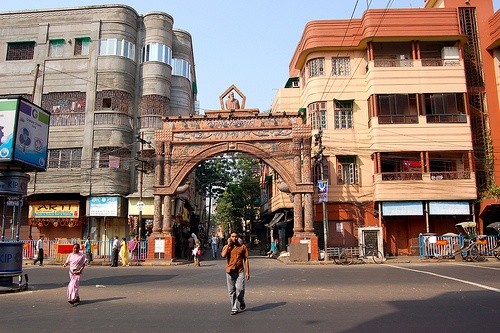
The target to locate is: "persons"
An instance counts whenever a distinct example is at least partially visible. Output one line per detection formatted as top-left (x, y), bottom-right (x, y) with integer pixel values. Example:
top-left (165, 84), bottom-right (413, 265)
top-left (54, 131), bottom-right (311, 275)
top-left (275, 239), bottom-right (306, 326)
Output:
top-left (63, 243), bottom-right (85, 305)
top-left (208, 232), bottom-right (227, 260)
top-left (120, 237), bottom-right (128, 267)
top-left (227, 92), bottom-right (239, 109)
top-left (181, 235), bottom-right (201, 267)
top-left (111, 235), bottom-right (120, 267)
top-left (33, 236), bottom-right (44, 266)
top-left (84, 236), bottom-right (91, 266)
top-left (132, 235), bottom-right (140, 266)
top-left (221, 230), bottom-right (250, 315)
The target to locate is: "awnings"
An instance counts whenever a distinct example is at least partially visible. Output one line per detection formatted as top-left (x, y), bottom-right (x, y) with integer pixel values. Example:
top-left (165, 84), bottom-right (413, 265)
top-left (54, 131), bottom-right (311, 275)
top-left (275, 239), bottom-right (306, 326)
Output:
top-left (265, 213), bottom-right (284, 227)
top-left (429, 202), bottom-right (470, 215)
top-left (272, 218), bottom-right (293, 228)
top-left (382, 202), bottom-right (423, 216)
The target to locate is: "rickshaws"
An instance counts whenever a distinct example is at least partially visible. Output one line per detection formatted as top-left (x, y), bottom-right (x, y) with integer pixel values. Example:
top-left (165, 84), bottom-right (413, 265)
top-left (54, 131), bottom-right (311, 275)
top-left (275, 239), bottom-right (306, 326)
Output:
top-left (479, 221), bottom-right (500, 260)
top-left (436, 221), bottom-right (489, 262)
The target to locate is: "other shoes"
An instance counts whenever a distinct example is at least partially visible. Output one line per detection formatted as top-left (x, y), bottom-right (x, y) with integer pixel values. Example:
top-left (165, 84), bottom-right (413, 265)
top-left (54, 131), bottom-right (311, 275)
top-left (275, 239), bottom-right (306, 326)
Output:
top-left (231, 308), bottom-right (239, 314)
top-left (68, 300), bottom-right (75, 305)
top-left (34, 261), bottom-right (36, 265)
top-left (240, 303), bottom-right (246, 310)
top-left (75, 296), bottom-right (80, 302)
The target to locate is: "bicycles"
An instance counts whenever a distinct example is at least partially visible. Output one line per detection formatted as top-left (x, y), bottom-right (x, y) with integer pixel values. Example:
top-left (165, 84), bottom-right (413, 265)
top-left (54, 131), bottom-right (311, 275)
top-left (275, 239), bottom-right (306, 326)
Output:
top-left (339, 243), bottom-right (384, 265)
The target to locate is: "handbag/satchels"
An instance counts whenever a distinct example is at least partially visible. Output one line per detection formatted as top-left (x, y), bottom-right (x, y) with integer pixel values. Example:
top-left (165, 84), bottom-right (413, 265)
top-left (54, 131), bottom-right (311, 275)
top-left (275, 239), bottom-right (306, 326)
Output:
top-left (192, 247), bottom-right (201, 256)
